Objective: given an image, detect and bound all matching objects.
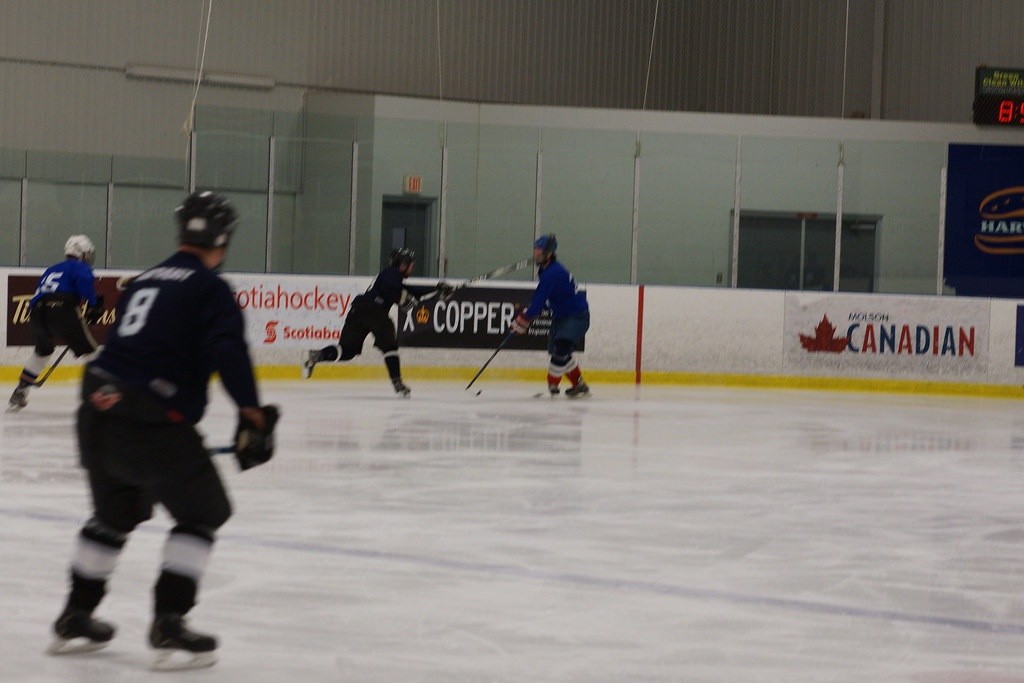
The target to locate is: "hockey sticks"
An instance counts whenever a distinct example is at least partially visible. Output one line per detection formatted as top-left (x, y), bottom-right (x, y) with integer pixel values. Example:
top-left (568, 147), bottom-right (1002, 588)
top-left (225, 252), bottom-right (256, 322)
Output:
top-left (203, 444), bottom-right (240, 454)
top-left (417, 230), bottom-right (555, 303)
top-left (464, 332), bottom-right (513, 396)
top-left (34, 344), bottom-right (72, 388)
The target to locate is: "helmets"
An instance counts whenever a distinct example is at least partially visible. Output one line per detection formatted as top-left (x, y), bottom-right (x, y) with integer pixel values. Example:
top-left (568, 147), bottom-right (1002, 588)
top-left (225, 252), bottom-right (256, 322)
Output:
top-left (535, 235), bottom-right (557, 249)
top-left (393, 248), bottom-right (415, 262)
top-left (176, 191), bottom-right (238, 247)
top-left (65, 234), bottom-right (95, 257)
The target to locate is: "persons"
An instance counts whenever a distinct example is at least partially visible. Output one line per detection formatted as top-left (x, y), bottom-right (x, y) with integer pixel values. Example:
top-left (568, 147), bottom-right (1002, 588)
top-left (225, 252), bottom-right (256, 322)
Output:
top-left (48, 191), bottom-right (281, 671)
top-left (5, 235), bottom-right (103, 413)
top-left (303, 248), bottom-right (429, 398)
top-left (510, 234), bottom-right (590, 399)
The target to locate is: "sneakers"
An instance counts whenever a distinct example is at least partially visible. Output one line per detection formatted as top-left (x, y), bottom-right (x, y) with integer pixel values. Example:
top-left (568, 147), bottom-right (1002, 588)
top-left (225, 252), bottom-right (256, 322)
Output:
top-left (564, 384), bottom-right (588, 397)
top-left (51, 606), bottom-right (113, 653)
top-left (392, 379), bottom-right (410, 398)
top-left (150, 616), bottom-right (217, 669)
top-left (302, 350), bottom-right (323, 378)
top-left (535, 386), bottom-right (560, 398)
top-left (7, 388), bottom-right (27, 412)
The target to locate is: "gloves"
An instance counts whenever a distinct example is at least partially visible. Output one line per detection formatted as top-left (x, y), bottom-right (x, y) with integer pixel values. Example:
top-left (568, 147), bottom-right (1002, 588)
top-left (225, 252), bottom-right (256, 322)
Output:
top-left (509, 312), bottom-right (533, 336)
top-left (235, 406), bottom-right (278, 469)
top-left (88, 296), bottom-right (103, 321)
